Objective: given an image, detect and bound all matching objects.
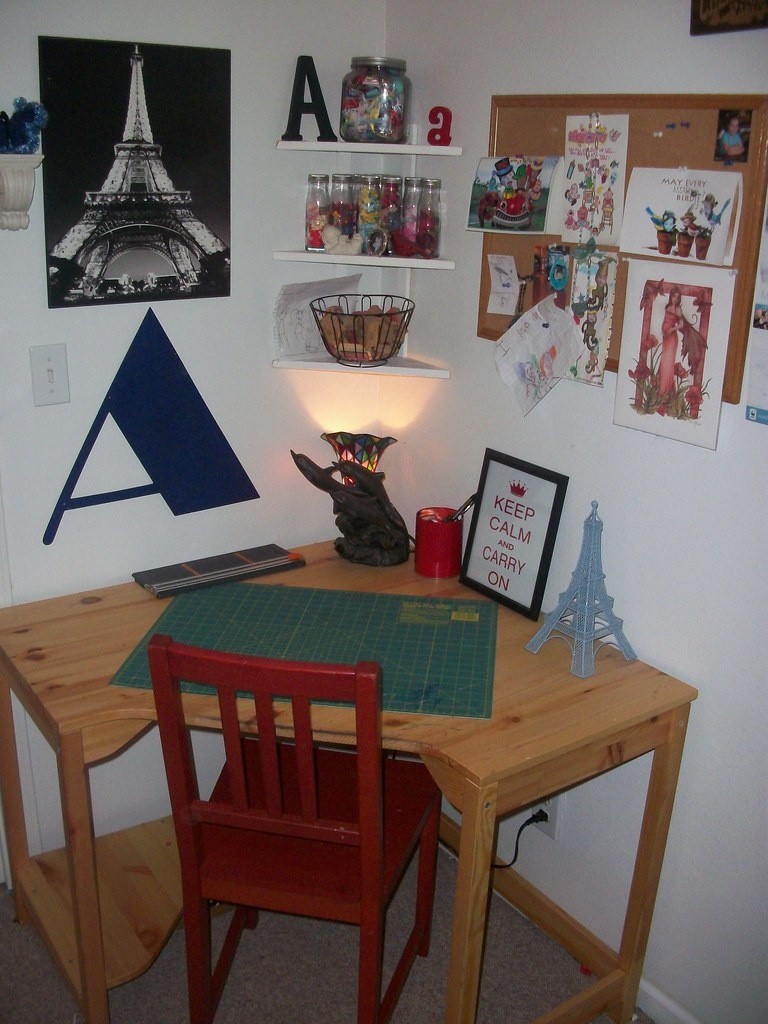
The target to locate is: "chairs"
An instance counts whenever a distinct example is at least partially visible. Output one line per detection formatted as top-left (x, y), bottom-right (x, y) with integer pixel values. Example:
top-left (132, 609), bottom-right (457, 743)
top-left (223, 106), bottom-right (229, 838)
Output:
top-left (146, 633), bottom-right (443, 1024)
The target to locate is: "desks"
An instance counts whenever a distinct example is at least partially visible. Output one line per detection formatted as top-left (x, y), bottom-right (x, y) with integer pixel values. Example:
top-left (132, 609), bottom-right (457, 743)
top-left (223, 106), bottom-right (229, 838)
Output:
top-left (0, 526), bottom-right (699, 1024)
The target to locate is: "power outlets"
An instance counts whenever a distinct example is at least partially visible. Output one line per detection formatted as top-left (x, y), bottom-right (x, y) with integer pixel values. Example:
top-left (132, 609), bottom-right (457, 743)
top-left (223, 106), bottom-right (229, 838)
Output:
top-left (531, 793), bottom-right (559, 840)
top-left (29, 342), bottom-right (71, 407)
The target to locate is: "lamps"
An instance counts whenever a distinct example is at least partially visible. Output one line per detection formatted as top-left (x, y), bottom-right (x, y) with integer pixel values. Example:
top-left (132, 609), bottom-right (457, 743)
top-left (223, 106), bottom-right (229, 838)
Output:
top-left (320, 432), bottom-right (398, 487)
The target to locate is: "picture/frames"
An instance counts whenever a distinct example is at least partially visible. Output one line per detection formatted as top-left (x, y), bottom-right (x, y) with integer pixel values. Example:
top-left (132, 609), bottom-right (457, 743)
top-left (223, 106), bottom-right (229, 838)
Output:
top-left (475, 93), bottom-right (768, 404)
top-left (690, 0), bottom-right (768, 36)
top-left (458, 445), bottom-right (571, 623)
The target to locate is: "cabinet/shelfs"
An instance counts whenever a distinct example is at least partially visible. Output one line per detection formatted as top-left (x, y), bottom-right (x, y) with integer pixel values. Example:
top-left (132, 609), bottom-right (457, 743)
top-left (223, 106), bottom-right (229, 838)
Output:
top-left (272, 113), bottom-right (459, 379)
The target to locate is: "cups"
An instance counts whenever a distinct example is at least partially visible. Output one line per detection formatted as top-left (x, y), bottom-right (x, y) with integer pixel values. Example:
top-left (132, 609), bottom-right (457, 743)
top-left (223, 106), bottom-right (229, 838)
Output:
top-left (677, 232), bottom-right (693, 257)
top-left (414, 507), bottom-right (464, 578)
top-left (657, 230), bottom-right (673, 254)
top-left (695, 237), bottom-right (711, 259)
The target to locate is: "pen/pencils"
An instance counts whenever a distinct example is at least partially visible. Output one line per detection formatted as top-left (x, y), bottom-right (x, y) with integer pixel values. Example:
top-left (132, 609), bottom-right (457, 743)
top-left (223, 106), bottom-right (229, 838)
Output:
top-left (452, 492), bottom-right (478, 522)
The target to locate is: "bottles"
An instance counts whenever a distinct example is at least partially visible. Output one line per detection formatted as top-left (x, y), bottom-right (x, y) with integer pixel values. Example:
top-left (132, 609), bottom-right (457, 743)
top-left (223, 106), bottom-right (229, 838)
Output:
top-left (403, 177), bottom-right (423, 243)
top-left (417, 179), bottom-right (441, 258)
top-left (339, 57), bottom-right (412, 143)
top-left (358, 176), bottom-right (380, 252)
top-left (351, 174), bottom-right (364, 234)
top-left (381, 176), bottom-right (402, 253)
top-left (332, 174), bottom-right (352, 238)
top-left (306, 174), bottom-right (330, 252)
